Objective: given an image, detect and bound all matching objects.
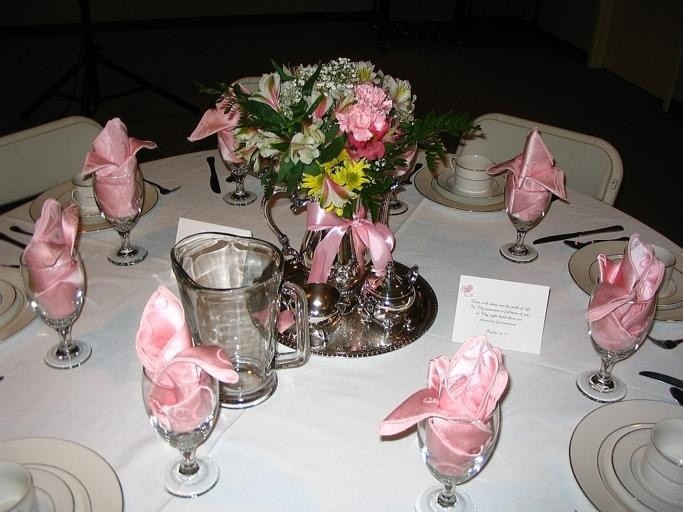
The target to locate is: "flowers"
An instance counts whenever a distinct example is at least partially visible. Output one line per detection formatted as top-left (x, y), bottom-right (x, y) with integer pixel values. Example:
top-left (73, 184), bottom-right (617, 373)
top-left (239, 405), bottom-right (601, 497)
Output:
top-left (233, 57), bottom-right (417, 206)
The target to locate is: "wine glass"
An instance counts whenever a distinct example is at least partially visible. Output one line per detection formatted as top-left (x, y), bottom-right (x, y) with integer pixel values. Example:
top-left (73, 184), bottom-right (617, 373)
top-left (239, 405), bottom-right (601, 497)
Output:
top-left (140, 365), bottom-right (221, 498)
top-left (498, 172), bottom-right (554, 264)
top-left (385, 137), bottom-right (419, 217)
top-left (289, 282), bottom-right (351, 350)
top-left (573, 276), bottom-right (658, 403)
top-left (18, 246), bottom-right (93, 372)
top-left (413, 401), bottom-right (501, 512)
top-left (91, 163), bottom-right (148, 267)
top-left (216, 128), bottom-right (260, 206)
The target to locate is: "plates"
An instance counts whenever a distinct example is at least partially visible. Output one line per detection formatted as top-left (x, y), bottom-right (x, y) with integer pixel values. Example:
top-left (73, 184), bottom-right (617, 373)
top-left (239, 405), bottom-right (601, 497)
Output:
top-left (413, 162), bottom-right (511, 213)
top-left (0, 264), bottom-right (37, 343)
top-left (568, 240), bottom-right (683, 322)
top-left (0, 435), bottom-right (124, 512)
top-left (28, 177), bottom-right (159, 233)
top-left (436, 168), bottom-right (507, 200)
top-left (24, 465), bottom-right (75, 512)
top-left (568, 398), bottom-right (683, 511)
top-left (587, 251), bottom-right (683, 310)
top-left (610, 415), bottom-right (683, 511)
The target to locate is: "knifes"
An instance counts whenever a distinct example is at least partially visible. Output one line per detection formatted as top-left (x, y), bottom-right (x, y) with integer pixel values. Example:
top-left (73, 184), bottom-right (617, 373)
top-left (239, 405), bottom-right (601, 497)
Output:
top-left (532, 225), bottom-right (623, 246)
top-left (206, 156), bottom-right (221, 195)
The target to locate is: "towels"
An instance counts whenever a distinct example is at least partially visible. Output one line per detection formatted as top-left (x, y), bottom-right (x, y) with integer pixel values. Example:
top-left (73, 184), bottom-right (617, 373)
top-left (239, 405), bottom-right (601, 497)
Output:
top-left (583, 231), bottom-right (666, 356)
top-left (485, 123), bottom-right (569, 224)
top-left (135, 283), bottom-right (239, 435)
top-left (20, 198), bottom-right (84, 322)
top-left (81, 117), bottom-right (158, 219)
top-left (187, 82), bottom-right (256, 166)
top-left (378, 334), bottom-right (509, 476)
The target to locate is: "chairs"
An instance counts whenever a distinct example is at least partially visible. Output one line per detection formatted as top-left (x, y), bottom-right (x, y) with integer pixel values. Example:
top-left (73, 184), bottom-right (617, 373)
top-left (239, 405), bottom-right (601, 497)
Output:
top-left (454, 111), bottom-right (624, 207)
top-left (0, 115), bottom-right (104, 214)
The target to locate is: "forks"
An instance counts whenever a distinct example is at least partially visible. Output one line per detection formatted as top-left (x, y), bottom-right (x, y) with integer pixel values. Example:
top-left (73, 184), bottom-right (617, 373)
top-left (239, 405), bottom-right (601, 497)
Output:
top-left (143, 177), bottom-right (182, 194)
top-left (647, 335), bottom-right (683, 350)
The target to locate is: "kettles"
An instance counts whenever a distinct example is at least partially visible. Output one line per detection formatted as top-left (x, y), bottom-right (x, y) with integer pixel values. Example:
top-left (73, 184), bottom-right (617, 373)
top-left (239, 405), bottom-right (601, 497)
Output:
top-left (259, 181), bottom-right (403, 293)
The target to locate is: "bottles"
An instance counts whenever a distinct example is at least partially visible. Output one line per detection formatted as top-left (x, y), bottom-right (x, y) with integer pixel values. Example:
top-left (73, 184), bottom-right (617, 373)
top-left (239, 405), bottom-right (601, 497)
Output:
top-left (358, 263), bottom-right (417, 326)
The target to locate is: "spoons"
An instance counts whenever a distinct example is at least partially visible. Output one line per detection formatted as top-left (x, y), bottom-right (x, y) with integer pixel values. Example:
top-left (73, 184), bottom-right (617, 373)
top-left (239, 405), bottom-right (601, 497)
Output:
top-left (399, 162), bottom-right (423, 186)
top-left (563, 236), bottom-right (631, 251)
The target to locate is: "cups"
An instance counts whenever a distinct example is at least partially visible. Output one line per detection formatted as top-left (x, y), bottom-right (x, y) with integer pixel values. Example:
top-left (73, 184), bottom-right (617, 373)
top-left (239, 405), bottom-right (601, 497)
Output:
top-left (0, 457), bottom-right (40, 512)
top-left (70, 170), bottom-right (100, 212)
top-left (168, 231), bottom-right (313, 410)
top-left (645, 244), bottom-right (677, 294)
top-left (449, 154), bottom-right (495, 196)
top-left (639, 419), bottom-right (683, 506)
